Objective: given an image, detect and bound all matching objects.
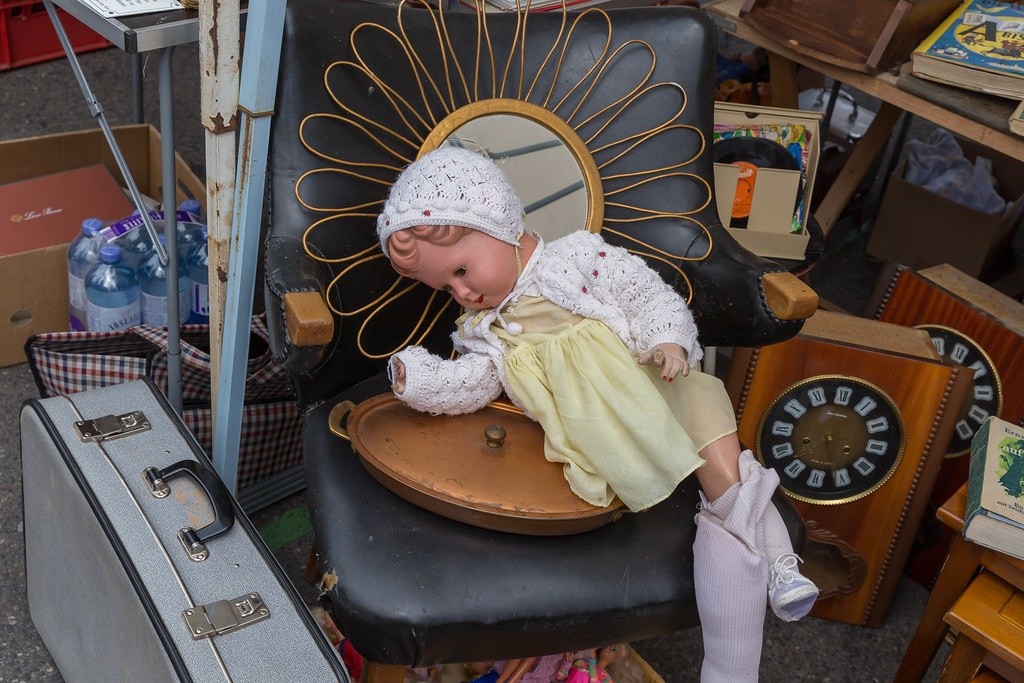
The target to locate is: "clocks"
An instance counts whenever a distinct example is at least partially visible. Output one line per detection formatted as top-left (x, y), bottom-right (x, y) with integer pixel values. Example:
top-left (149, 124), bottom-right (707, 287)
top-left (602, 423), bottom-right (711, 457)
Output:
top-left (754, 373), bottom-right (906, 506)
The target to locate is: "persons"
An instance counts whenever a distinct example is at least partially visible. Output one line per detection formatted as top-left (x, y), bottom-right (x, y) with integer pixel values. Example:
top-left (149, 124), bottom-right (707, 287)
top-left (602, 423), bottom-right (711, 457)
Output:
top-left (378, 147), bottom-right (820, 683)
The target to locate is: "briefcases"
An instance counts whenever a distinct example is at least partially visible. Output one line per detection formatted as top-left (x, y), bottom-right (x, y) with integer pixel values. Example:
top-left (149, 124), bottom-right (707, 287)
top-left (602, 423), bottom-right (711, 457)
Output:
top-left (20, 376), bottom-right (351, 683)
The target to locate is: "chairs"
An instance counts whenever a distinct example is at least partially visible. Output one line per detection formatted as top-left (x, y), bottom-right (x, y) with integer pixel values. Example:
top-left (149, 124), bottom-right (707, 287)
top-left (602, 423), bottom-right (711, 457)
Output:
top-left (257, 6), bottom-right (821, 661)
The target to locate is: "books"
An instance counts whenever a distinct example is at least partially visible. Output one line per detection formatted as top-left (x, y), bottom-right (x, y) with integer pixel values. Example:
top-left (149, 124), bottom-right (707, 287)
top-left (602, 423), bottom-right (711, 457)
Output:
top-left (961, 416), bottom-right (1024, 558)
top-left (911, 0), bottom-right (1024, 135)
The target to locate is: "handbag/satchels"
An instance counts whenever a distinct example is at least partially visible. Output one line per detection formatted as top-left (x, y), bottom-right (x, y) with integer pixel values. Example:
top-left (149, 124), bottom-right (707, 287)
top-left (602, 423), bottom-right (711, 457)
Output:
top-left (23, 313), bottom-right (305, 491)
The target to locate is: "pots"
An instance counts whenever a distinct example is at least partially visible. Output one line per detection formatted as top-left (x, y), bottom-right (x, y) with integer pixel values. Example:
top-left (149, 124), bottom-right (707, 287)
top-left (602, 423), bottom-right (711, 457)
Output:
top-left (328, 392), bottom-right (652, 536)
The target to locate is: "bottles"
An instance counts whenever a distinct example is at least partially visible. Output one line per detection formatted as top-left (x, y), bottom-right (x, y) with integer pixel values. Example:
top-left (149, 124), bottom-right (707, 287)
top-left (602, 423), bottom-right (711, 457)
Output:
top-left (67, 199), bottom-right (207, 333)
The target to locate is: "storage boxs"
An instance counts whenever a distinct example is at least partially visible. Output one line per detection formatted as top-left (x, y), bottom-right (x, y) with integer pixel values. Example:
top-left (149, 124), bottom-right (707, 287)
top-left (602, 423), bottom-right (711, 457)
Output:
top-left (868, 140), bottom-right (1024, 282)
top-left (0, 123), bottom-right (211, 372)
